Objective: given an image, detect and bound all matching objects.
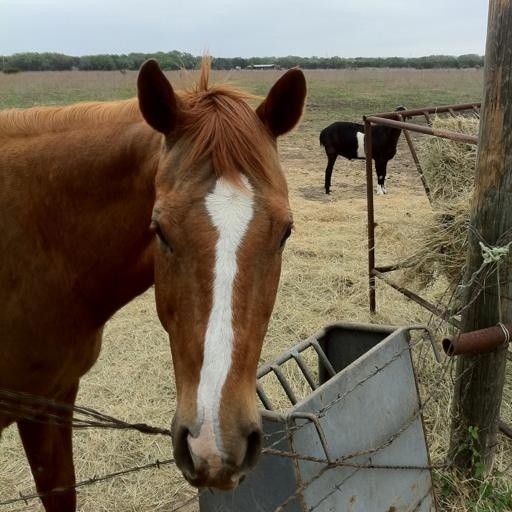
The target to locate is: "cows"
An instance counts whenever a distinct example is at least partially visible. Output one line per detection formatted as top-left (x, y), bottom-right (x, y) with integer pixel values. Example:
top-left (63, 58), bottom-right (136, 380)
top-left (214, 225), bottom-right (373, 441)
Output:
top-left (319, 107), bottom-right (412, 196)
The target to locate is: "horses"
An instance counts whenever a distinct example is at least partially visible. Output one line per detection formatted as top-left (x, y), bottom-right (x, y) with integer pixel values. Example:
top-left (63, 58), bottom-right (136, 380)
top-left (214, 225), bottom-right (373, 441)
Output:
top-left (0, 47), bottom-right (306, 512)
